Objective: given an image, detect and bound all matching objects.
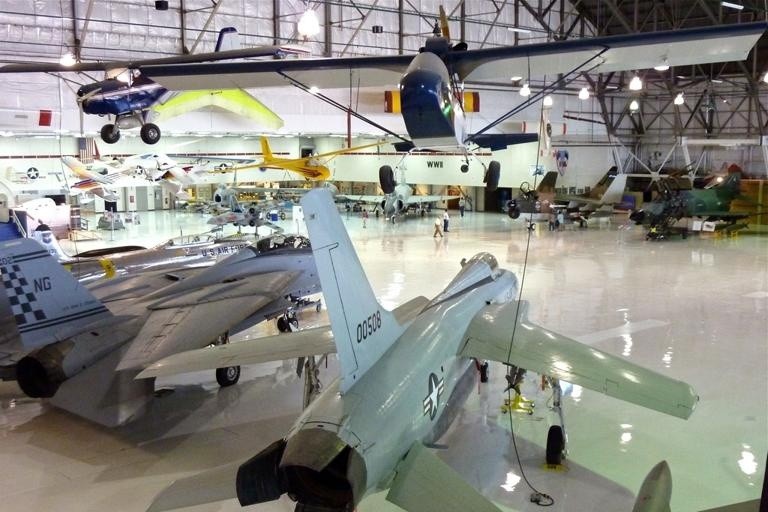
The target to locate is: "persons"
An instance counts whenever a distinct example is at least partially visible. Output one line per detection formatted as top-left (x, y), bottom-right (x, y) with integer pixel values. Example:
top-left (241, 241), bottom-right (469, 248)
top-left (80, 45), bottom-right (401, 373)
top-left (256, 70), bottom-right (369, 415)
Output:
top-left (547, 211), bottom-right (555, 231)
top-left (645, 223), bottom-right (658, 240)
top-left (442, 209), bottom-right (450, 233)
top-left (432, 215), bottom-right (444, 238)
top-left (458, 197), bottom-right (466, 218)
top-left (360, 208), bottom-right (369, 230)
top-left (556, 210), bottom-right (564, 232)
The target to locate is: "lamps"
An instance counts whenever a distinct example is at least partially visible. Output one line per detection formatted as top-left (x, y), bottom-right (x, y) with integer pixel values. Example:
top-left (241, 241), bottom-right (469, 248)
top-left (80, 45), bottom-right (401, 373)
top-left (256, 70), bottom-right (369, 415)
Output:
top-left (509, 62), bottom-right (686, 112)
top-left (297, 0), bottom-right (319, 38)
top-left (59, 43), bottom-right (78, 68)
